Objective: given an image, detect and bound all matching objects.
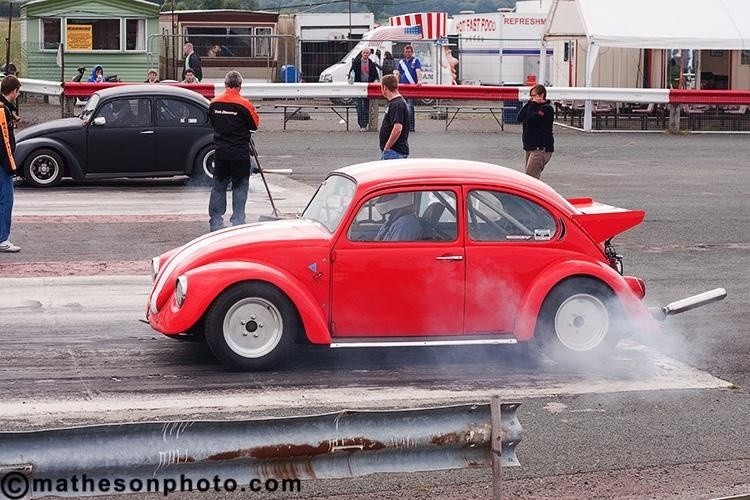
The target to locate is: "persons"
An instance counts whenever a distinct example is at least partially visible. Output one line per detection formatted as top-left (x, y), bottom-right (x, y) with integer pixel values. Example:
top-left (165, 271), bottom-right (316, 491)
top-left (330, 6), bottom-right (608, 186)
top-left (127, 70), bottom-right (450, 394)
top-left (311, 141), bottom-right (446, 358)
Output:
top-left (517, 84), bottom-right (554, 178)
top-left (207, 45), bottom-right (219, 58)
top-left (209, 71), bottom-right (259, 231)
top-left (143, 69), bottom-right (160, 84)
top-left (374, 189), bottom-right (423, 239)
top-left (375, 51), bottom-right (395, 76)
top-left (397, 45), bottom-right (422, 132)
top-left (87, 64), bottom-right (106, 83)
top-left (378, 74), bottom-right (410, 160)
top-left (182, 42), bottom-right (202, 82)
top-left (0, 74), bottom-right (21, 253)
top-left (179, 69), bottom-right (199, 84)
top-left (349, 46), bottom-right (378, 132)
top-left (442, 38), bottom-right (459, 85)
top-left (368, 49), bottom-right (380, 74)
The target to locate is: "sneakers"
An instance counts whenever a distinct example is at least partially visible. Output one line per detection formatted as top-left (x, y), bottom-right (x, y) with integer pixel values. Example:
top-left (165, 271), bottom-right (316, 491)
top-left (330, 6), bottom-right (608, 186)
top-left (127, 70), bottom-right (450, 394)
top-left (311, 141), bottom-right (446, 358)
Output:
top-left (0, 240), bottom-right (21, 253)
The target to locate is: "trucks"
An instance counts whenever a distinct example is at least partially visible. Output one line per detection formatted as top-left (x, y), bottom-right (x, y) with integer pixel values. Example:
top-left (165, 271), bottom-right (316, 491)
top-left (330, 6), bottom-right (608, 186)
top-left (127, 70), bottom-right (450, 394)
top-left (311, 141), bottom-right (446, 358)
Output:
top-left (321, 10), bottom-right (464, 107)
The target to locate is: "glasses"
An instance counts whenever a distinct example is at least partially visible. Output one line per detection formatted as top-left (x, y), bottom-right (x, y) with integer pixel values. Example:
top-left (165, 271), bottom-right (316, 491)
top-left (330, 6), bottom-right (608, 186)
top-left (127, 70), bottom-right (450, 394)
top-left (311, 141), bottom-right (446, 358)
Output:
top-left (529, 93), bottom-right (538, 98)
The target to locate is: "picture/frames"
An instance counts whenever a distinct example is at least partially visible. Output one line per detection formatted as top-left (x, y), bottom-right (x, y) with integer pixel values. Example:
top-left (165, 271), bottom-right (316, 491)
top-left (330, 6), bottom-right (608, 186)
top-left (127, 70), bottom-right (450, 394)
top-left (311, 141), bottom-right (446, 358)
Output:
top-left (709, 50), bottom-right (723, 57)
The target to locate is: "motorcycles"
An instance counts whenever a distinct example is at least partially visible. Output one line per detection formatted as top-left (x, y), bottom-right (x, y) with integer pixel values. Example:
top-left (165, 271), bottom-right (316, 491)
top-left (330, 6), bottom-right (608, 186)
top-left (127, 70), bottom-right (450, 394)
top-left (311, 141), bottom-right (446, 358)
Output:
top-left (58, 65), bottom-right (122, 106)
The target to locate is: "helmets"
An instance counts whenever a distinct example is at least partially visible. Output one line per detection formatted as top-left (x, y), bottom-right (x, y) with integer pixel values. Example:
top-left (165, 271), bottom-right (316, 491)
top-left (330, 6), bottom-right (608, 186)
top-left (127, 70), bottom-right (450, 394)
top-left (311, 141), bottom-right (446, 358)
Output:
top-left (375, 192), bottom-right (414, 216)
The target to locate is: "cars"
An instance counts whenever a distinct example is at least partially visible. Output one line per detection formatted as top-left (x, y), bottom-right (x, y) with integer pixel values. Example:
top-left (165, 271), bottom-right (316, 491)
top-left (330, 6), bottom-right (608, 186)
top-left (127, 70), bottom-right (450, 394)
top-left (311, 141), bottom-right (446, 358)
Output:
top-left (12, 82), bottom-right (296, 188)
top-left (145, 156), bottom-right (728, 376)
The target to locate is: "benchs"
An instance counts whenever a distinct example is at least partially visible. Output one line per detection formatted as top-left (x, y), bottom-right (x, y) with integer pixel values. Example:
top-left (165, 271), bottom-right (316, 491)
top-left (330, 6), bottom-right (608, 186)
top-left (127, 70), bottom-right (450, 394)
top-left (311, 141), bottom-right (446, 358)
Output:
top-left (272, 104), bottom-right (358, 132)
top-left (431, 107), bottom-right (517, 131)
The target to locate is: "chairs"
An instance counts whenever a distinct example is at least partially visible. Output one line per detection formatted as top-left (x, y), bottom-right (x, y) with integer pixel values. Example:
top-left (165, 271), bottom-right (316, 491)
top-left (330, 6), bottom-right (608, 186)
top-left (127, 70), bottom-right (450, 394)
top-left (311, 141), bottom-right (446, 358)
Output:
top-left (552, 105), bottom-right (750, 138)
